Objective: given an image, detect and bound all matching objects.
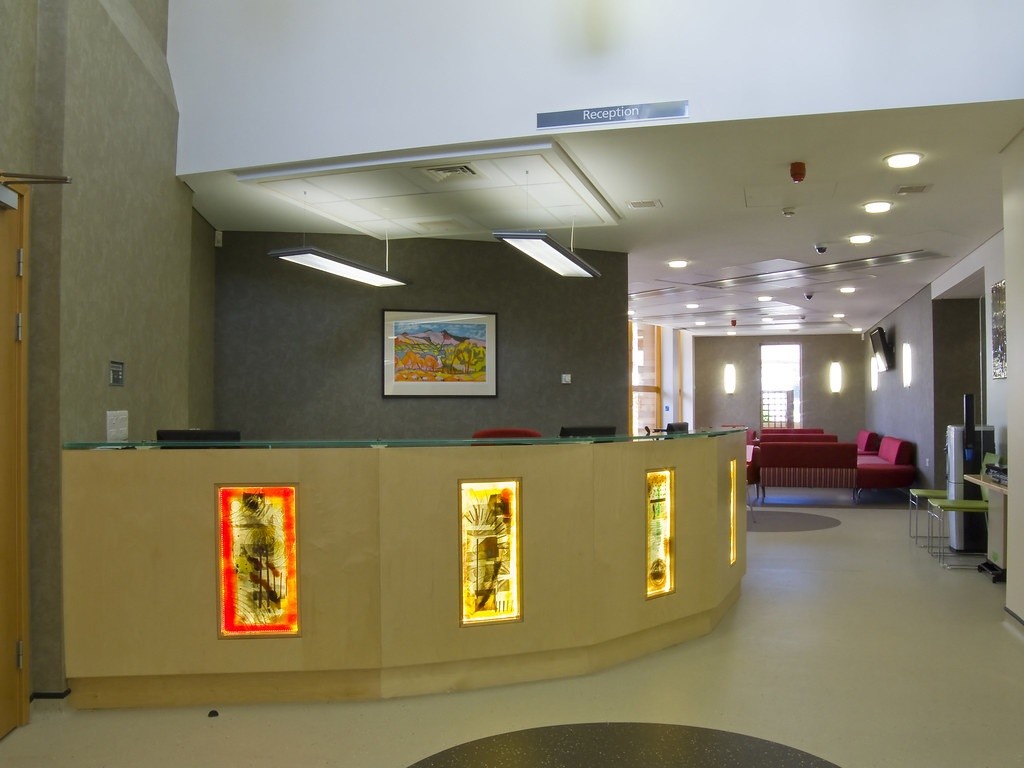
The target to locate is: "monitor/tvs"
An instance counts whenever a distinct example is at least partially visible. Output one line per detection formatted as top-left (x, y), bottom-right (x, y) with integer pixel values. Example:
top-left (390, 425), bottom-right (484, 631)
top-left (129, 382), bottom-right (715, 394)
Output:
top-left (667, 422), bottom-right (689, 430)
top-left (869, 327), bottom-right (893, 372)
top-left (559, 426), bottom-right (617, 443)
top-left (156, 430), bottom-right (242, 449)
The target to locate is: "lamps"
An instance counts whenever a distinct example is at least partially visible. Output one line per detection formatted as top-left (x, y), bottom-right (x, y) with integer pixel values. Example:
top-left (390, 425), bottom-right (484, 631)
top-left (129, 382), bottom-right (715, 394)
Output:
top-left (870, 355), bottom-right (879, 392)
top-left (724, 360), bottom-right (736, 395)
top-left (829, 360), bottom-right (842, 394)
top-left (266, 189), bottom-right (413, 288)
top-left (902, 341), bottom-right (912, 387)
top-left (491, 156), bottom-right (603, 279)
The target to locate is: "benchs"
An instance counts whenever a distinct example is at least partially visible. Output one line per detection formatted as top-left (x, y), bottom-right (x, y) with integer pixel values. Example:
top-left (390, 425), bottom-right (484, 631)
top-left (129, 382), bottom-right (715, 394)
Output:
top-left (757, 427), bottom-right (858, 501)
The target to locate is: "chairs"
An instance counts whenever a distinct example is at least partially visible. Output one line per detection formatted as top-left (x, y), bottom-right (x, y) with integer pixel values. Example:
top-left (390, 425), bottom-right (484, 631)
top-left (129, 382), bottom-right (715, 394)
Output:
top-left (721, 424), bottom-right (761, 500)
top-left (856, 430), bottom-right (918, 498)
top-left (909, 484), bottom-right (950, 547)
top-left (927, 452), bottom-right (1001, 569)
top-left (667, 422), bottom-right (689, 431)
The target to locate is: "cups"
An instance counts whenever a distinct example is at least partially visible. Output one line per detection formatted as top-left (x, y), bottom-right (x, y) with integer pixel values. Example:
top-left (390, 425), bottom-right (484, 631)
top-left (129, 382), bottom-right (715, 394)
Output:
top-left (700, 427), bottom-right (706, 432)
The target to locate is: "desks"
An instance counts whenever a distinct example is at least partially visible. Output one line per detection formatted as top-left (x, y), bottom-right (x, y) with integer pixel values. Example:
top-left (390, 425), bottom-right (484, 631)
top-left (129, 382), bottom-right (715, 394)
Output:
top-left (961, 470), bottom-right (1007, 583)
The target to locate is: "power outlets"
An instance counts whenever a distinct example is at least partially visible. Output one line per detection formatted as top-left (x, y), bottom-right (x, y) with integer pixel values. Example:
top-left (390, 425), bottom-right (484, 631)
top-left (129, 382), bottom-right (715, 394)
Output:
top-left (562, 374), bottom-right (572, 384)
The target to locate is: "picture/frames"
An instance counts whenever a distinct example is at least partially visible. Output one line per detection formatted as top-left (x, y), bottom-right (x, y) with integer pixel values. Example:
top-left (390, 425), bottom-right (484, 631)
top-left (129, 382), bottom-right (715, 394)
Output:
top-left (380, 308), bottom-right (501, 401)
